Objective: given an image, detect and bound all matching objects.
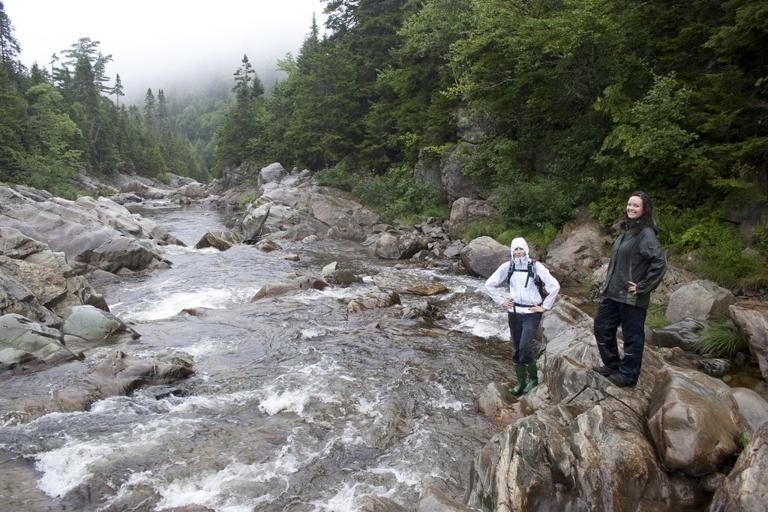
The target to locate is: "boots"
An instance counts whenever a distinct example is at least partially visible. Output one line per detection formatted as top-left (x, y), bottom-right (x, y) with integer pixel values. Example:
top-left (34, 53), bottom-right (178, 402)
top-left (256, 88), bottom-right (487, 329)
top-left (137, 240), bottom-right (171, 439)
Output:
top-left (523, 361), bottom-right (539, 393)
top-left (506, 360), bottom-right (527, 396)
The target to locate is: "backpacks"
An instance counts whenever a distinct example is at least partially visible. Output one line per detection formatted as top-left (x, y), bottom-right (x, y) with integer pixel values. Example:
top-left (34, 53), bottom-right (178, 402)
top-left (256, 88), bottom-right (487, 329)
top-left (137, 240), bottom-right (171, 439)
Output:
top-left (506, 258), bottom-right (551, 307)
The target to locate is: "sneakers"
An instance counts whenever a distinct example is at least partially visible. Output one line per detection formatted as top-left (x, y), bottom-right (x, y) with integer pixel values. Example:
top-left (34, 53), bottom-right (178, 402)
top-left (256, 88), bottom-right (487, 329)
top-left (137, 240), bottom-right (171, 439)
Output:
top-left (608, 369), bottom-right (639, 388)
top-left (591, 362), bottom-right (619, 376)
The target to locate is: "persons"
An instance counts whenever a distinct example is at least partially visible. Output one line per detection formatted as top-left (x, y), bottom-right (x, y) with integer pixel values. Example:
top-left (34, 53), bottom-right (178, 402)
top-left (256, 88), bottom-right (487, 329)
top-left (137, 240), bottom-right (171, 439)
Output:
top-left (485, 236), bottom-right (561, 397)
top-left (592, 190), bottom-right (669, 389)
